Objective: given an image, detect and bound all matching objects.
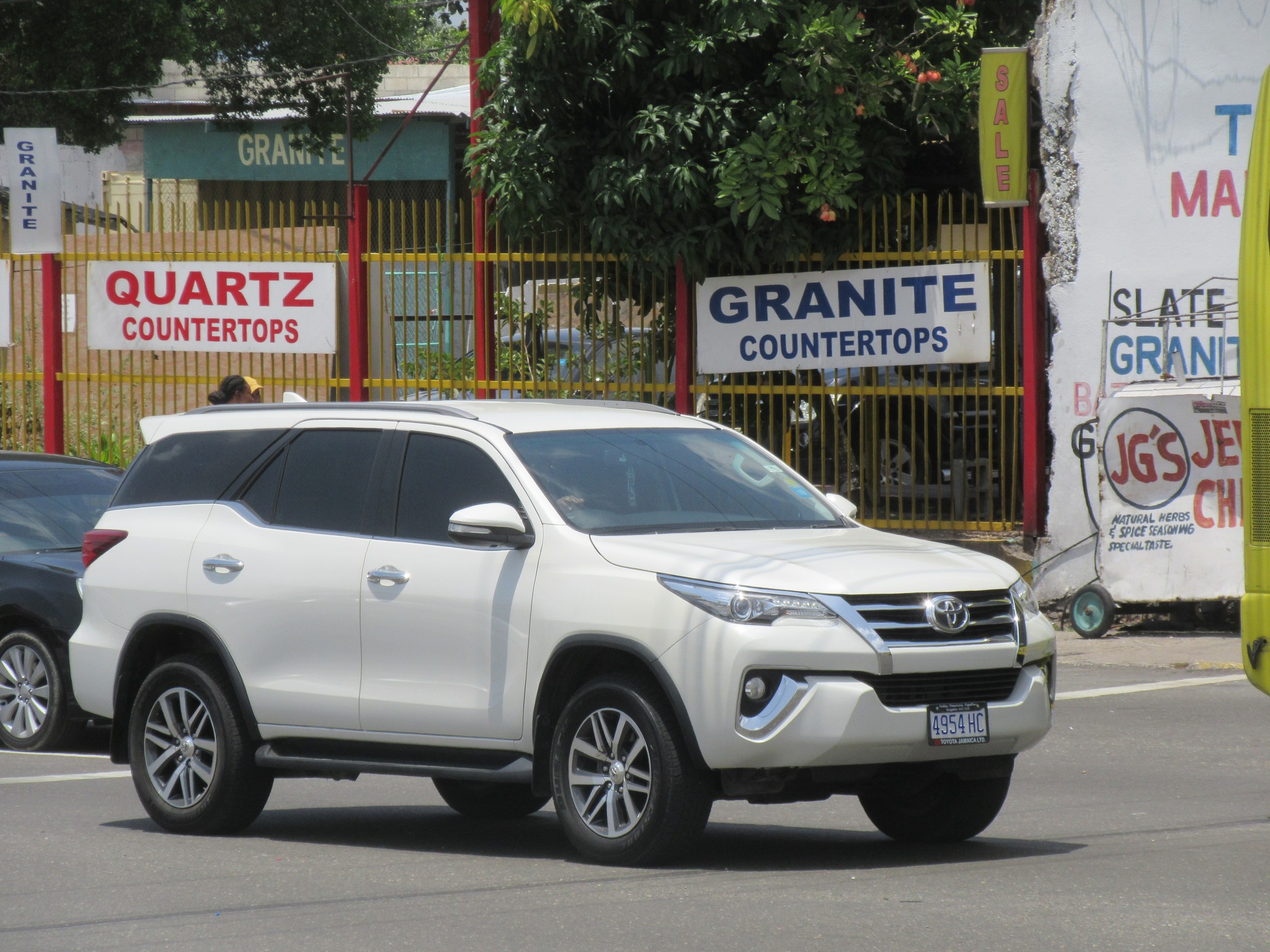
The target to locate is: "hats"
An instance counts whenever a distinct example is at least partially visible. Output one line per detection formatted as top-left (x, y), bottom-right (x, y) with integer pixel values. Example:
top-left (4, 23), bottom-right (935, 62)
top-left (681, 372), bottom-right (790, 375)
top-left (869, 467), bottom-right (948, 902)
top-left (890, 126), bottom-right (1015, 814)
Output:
top-left (243, 376), bottom-right (264, 393)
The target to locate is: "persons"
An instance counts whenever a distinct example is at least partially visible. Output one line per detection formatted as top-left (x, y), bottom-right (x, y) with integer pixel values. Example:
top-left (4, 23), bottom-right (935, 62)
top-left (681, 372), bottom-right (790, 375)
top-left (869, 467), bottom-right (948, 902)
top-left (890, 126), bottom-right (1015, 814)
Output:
top-left (243, 376), bottom-right (264, 403)
top-left (557, 496), bottom-right (584, 514)
top-left (207, 375), bottom-right (254, 405)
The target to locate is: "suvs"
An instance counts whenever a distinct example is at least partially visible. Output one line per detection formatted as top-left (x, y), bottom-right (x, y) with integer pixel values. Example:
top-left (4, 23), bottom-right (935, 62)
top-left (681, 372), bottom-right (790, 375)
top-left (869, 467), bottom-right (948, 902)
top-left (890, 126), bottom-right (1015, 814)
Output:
top-left (70, 391), bottom-right (1058, 868)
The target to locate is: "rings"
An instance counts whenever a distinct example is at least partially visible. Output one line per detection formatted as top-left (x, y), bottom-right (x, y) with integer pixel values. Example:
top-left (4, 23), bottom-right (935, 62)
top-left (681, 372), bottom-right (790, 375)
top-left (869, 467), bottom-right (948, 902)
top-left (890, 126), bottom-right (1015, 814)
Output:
top-left (566, 498), bottom-right (570, 501)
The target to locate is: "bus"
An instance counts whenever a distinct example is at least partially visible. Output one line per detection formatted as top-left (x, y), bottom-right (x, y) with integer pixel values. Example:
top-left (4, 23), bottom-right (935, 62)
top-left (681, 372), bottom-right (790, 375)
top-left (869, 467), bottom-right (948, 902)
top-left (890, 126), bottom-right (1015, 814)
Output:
top-left (1240, 72), bottom-right (1270, 695)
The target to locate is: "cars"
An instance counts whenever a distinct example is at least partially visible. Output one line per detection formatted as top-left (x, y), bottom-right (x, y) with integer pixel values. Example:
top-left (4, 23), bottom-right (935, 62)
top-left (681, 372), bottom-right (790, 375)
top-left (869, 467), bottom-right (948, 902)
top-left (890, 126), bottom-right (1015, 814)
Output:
top-left (0, 451), bottom-right (131, 752)
top-left (377, 265), bottom-right (1022, 496)
